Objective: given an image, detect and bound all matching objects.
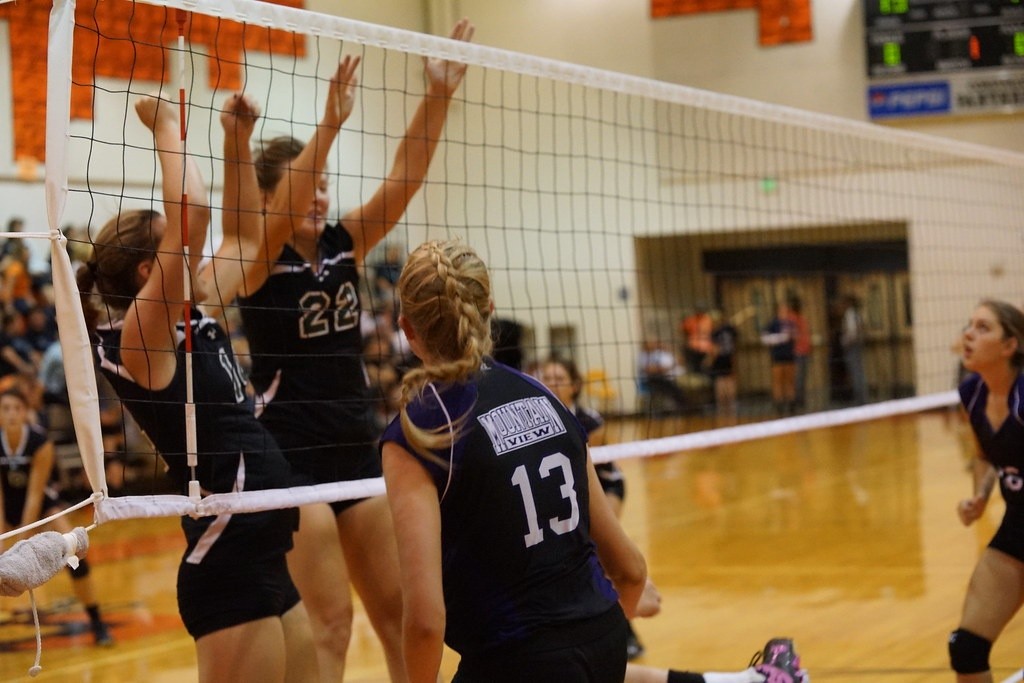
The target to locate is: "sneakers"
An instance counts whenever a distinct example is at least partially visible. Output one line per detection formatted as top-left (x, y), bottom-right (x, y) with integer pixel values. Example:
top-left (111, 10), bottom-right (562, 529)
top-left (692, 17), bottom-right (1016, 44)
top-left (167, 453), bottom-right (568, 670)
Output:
top-left (749, 637), bottom-right (810, 683)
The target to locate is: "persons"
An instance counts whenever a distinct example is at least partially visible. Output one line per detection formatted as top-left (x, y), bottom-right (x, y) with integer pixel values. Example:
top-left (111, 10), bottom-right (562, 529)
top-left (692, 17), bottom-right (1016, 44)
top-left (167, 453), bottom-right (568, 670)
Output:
top-left (683, 304), bottom-right (738, 419)
top-left (546, 357), bottom-right (663, 667)
top-left (2, 13), bottom-right (480, 683)
top-left (759, 294), bottom-right (808, 417)
top-left (640, 335), bottom-right (716, 426)
top-left (379, 236), bottom-right (811, 683)
top-left (835, 296), bottom-right (869, 411)
top-left (945, 299), bottom-right (1023, 683)
